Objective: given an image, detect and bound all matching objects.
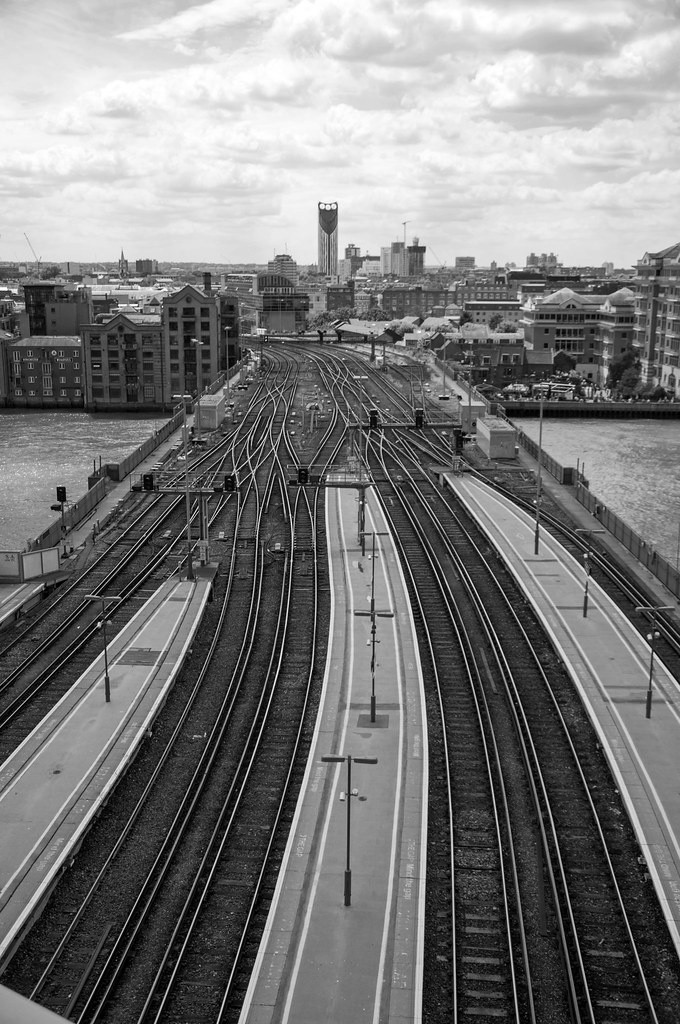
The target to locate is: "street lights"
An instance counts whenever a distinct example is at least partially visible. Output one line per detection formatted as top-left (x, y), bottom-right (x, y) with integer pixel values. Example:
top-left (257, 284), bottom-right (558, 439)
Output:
top-left (321, 755), bottom-right (378, 906)
top-left (635, 605), bottom-right (676, 719)
top-left (352, 482), bottom-right (376, 557)
top-left (353, 610), bottom-right (395, 724)
top-left (85, 595), bottom-right (122, 702)
top-left (172, 393), bottom-right (193, 581)
top-left (358, 532), bottom-right (390, 622)
top-left (575, 528), bottom-right (606, 617)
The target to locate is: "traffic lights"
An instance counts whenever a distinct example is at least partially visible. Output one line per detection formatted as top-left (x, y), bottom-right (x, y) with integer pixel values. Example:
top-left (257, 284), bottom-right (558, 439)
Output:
top-left (452, 427), bottom-right (464, 448)
top-left (143, 474), bottom-right (155, 491)
top-left (414, 407), bottom-right (424, 428)
top-left (369, 409), bottom-right (379, 429)
top-left (298, 469), bottom-right (309, 484)
top-left (57, 486), bottom-right (66, 501)
top-left (224, 476), bottom-right (236, 491)
